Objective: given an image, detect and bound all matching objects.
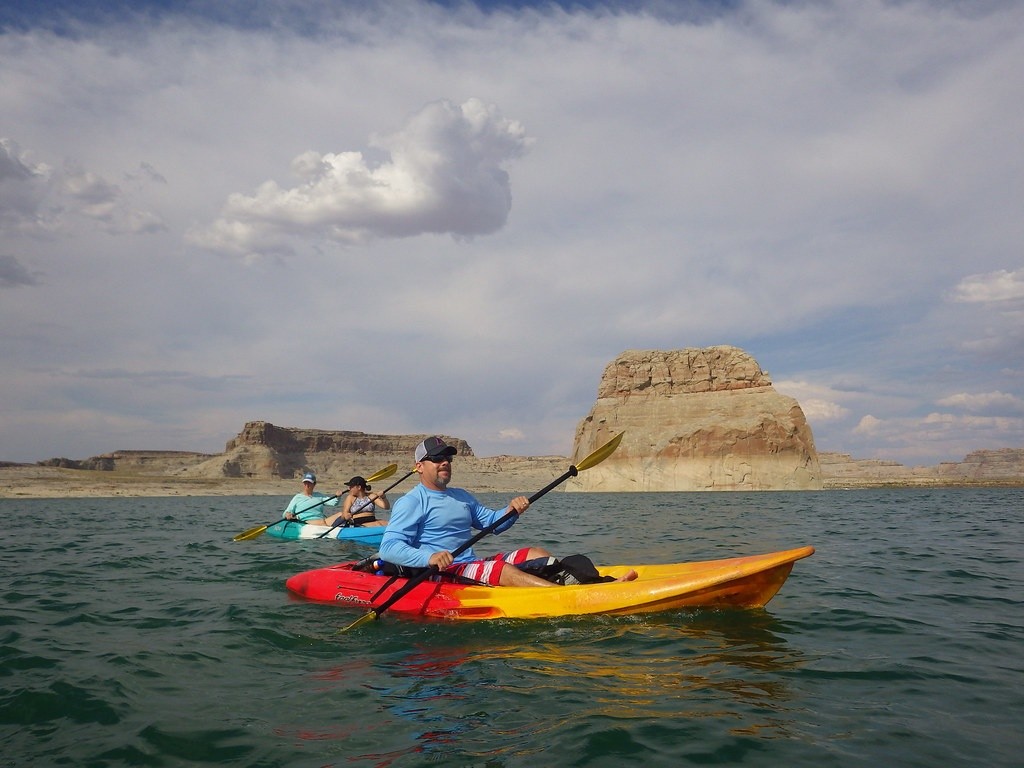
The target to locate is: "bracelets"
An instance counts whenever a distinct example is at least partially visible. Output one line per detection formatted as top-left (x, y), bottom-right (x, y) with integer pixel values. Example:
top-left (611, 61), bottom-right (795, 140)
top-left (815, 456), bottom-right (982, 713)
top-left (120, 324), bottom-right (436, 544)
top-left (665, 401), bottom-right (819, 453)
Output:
top-left (381, 496), bottom-right (387, 500)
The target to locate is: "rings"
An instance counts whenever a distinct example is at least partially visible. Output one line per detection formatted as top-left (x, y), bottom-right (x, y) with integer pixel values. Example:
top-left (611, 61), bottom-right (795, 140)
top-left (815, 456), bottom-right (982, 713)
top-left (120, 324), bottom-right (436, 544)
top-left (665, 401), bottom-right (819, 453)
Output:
top-left (523, 503), bottom-right (526, 505)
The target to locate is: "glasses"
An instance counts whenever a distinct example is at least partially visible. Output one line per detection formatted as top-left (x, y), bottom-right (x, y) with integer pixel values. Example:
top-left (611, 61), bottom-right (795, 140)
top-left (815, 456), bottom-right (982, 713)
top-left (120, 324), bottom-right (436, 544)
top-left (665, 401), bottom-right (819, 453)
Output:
top-left (420, 453), bottom-right (453, 463)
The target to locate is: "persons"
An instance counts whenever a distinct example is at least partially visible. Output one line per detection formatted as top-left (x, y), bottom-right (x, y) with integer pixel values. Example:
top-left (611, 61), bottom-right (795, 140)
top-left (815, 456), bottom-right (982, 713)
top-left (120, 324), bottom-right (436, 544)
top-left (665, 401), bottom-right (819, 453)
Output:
top-left (379, 437), bottom-right (640, 588)
top-left (342, 476), bottom-right (390, 527)
top-left (281, 472), bottom-right (345, 526)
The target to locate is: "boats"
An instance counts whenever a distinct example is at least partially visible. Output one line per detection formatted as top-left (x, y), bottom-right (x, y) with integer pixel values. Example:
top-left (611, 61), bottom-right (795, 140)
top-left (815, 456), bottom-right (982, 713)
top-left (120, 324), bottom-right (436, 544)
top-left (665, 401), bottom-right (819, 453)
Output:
top-left (267, 519), bottom-right (387, 544)
top-left (286, 547), bottom-right (816, 621)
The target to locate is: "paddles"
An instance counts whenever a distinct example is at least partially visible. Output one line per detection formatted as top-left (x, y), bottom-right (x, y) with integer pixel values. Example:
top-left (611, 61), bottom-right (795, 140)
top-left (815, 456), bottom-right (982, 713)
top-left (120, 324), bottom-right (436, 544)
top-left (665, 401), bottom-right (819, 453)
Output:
top-left (231, 462), bottom-right (398, 541)
top-left (339, 429), bottom-right (625, 631)
top-left (318, 468), bottom-right (418, 539)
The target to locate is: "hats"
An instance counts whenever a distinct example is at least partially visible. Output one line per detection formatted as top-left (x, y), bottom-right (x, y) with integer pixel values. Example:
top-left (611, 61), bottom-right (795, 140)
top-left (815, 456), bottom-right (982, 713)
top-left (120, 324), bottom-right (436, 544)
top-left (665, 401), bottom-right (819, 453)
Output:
top-left (344, 476), bottom-right (366, 486)
top-left (414, 437), bottom-right (457, 463)
top-left (302, 474), bottom-right (316, 483)
